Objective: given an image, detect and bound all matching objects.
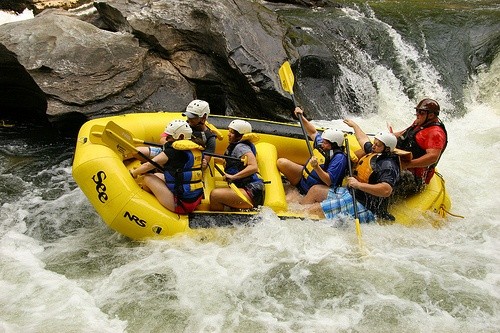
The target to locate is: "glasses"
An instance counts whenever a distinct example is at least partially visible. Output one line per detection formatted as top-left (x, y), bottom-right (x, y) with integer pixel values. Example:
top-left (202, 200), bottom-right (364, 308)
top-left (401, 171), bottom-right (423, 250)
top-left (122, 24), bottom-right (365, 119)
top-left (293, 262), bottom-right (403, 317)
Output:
top-left (416, 108), bottom-right (431, 114)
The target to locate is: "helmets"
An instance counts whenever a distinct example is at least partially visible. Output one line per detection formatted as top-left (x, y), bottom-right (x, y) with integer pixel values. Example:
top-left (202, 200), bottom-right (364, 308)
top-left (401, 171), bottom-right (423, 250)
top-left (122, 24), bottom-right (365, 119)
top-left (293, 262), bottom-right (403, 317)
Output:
top-left (228, 119), bottom-right (252, 133)
top-left (161, 118), bottom-right (192, 138)
top-left (414, 98), bottom-right (440, 115)
top-left (182, 99), bottom-right (210, 119)
top-left (321, 127), bottom-right (344, 146)
top-left (374, 131), bottom-right (397, 152)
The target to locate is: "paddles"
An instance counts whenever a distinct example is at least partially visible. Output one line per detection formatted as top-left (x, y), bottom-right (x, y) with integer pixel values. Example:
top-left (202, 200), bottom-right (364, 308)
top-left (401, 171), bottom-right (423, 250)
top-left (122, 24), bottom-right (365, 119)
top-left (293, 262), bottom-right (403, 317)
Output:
top-left (344, 133), bottom-right (363, 246)
top-left (100, 121), bottom-right (166, 174)
top-left (213, 163), bottom-right (253, 207)
top-left (89, 124), bottom-right (248, 167)
top-left (278, 61), bottom-right (313, 157)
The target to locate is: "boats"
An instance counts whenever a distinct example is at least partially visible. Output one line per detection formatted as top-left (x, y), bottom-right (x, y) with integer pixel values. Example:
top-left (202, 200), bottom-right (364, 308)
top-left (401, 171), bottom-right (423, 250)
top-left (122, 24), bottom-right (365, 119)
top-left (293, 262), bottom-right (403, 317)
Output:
top-left (71, 110), bottom-right (453, 245)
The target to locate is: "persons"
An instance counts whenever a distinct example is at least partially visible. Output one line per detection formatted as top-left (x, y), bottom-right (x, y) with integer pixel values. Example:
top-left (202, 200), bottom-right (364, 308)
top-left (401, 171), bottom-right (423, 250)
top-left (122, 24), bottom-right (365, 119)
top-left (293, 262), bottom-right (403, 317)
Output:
top-left (129, 100), bottom-right (265, 215)
top-left (277, 99), bottom-right (448, 224)
